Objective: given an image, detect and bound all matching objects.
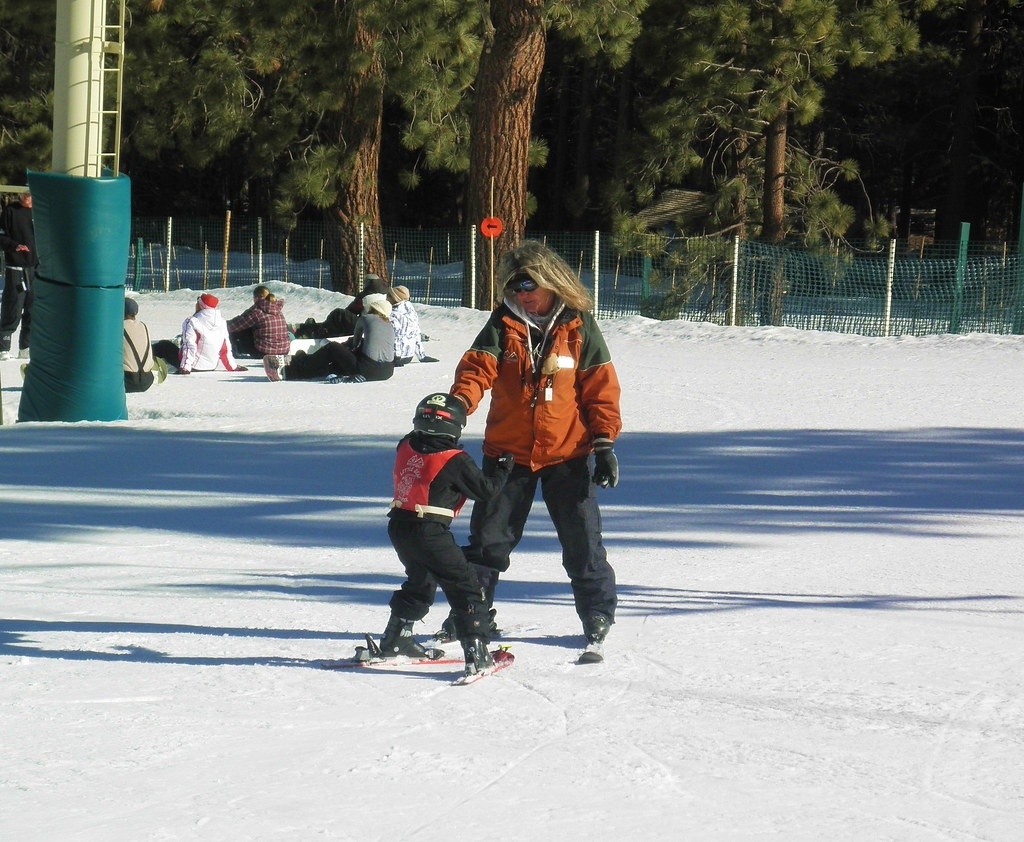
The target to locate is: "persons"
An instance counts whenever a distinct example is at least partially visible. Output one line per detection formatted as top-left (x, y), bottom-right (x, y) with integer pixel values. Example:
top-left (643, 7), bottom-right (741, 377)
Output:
top-left (0, 188), bottom-right (38, 361)
top-left (152, 294), bottom-right (250, 375)
top-left (261, 273), bottom-right (440, 384)
top-left (120, 297), bottom-right (155, 392)
top-left (382, 393), bottom-right (498, 676)
top-left (224, 287), bottom-right (291, 359)
top-left (442, 241), bottom-right (623, 643)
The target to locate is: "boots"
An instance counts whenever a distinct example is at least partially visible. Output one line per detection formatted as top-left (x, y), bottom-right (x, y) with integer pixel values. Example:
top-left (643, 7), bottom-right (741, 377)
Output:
top-left (461, 635), bottom-right (491, 674)
top-left (378, 615), bottom-right (443, 659)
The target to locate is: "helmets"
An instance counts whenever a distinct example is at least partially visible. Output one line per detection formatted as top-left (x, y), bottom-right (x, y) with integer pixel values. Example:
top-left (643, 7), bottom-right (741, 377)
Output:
top-left (413, 392), bottom-right (467, 440)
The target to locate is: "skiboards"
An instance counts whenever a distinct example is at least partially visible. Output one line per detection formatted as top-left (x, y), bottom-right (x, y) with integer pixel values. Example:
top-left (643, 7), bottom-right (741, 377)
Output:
top-left (320, 647), bottom-right (515, 685)
top-left (417, 622), bottom-right (604, 665)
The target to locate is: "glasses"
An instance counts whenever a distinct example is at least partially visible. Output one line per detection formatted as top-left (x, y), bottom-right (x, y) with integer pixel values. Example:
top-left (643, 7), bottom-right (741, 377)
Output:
top-left (506, 278), bottom-right (541, 293)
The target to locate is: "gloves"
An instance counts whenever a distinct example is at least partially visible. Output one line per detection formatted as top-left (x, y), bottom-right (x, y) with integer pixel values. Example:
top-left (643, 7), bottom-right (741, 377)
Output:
top-left (175, 369), bottom-right (190, 375)
top-left (420, 356), bottom-right (438, 362)
top-left (235, 365), bottom-right (247, 372)
top-left (495, 453), bottom-right (515, 474)
top-left (591, 443), bottom-right (618, 489)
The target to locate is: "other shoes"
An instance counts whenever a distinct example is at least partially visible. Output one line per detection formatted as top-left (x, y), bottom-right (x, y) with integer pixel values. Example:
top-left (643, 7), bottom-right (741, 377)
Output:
top-left (264, 354), bottom-right (284, 383)
top-left (1, 350), bottom-right (8, 361)
top-left (582, 615), bottom-right (609, 639)
top-left (296, 350), bottom-right (308, 375)
top-left (442, 609), bottom-right (497, 633)
top-left (18, 349), bottom-right (31, 359)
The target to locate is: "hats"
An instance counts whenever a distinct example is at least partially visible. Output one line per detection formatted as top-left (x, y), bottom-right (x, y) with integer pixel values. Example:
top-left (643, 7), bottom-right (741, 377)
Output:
top-left (388, 284), bottom-right (410, 303)
top-left (370, 299), bottom-right (393, 320)
top-left (15, 176), bottom-right (29, 186)
top-left (124, 296), bottom-right (138, 315)
top-left (199, 292), bottom-right (218, 309)
